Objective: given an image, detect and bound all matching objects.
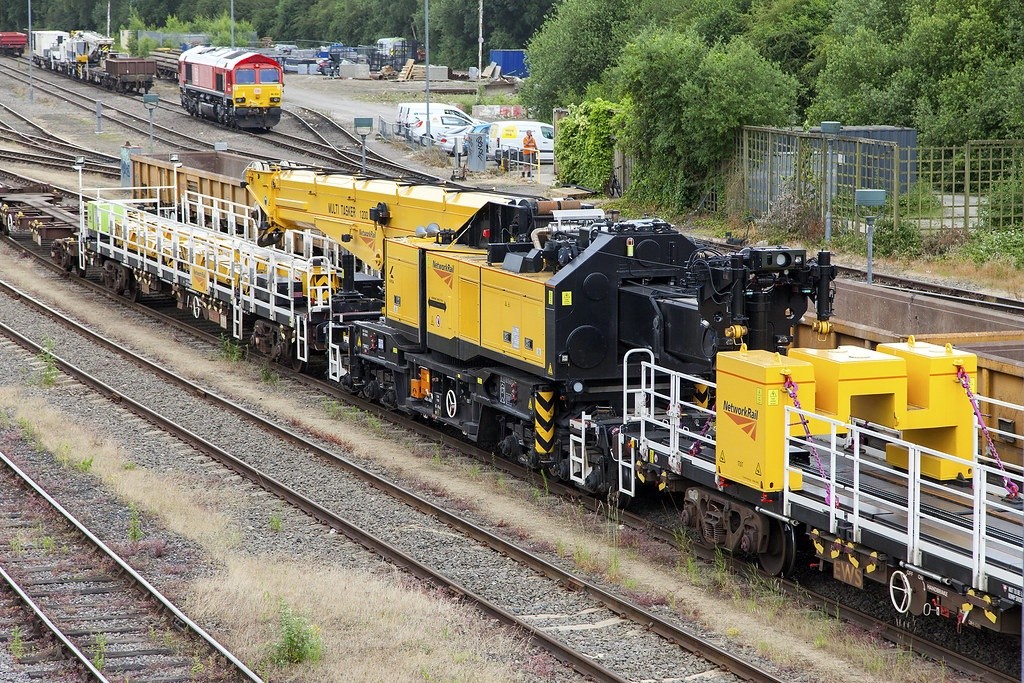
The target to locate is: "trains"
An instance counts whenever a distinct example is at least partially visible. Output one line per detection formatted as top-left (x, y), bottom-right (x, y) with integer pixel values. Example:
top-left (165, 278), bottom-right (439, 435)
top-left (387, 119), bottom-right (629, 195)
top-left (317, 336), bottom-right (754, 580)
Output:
top-left (179, 44), bottom-right (285, 131)
top-left (69, 142), bottom-right (1024, 637)
top-left (32, 28), bottom-right (156, 95)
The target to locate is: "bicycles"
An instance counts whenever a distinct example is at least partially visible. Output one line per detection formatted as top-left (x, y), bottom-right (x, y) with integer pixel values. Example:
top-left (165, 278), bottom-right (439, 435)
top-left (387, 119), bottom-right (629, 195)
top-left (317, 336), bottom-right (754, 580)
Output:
top-left (610, 165), bottom-right (623, 198)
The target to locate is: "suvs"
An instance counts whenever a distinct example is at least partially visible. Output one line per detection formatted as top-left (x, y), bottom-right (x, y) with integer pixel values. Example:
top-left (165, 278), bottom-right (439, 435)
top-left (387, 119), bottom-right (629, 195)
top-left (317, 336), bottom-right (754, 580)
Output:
top-left (409, 113), bottom-right (474, 146)
top-left (316, 58), bottom-right (357, 76)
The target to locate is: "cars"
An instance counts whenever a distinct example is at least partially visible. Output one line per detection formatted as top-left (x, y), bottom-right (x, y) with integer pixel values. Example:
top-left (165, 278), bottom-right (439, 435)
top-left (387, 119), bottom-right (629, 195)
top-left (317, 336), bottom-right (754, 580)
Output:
top-left (436, 125), bottom-right (474, 156)
top-left (463, 124), bottom-right (490, 152)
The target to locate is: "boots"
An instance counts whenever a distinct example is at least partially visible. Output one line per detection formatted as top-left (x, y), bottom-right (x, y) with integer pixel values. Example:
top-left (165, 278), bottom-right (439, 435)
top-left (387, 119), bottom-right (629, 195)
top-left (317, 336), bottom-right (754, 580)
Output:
top-left (522, 172), bottom-right (525, 177)
top-left (527, 172), bottom-right (534, 177)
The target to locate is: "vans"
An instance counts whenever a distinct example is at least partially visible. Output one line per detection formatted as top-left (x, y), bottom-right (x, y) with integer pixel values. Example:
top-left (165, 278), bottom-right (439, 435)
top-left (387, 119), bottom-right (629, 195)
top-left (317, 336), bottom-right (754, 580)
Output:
top-left (404, 106), bottom-right (483, 138)
top-left (398, 101), bottom-right (448, 135)
top-left (488, 120), bottom-right (554, 171)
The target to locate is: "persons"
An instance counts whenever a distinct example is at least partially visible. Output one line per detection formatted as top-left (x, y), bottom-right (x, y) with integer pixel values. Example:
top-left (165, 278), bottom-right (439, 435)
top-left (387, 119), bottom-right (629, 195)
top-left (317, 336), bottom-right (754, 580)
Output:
top-left (522, 130), bottom-right (540, 177)
